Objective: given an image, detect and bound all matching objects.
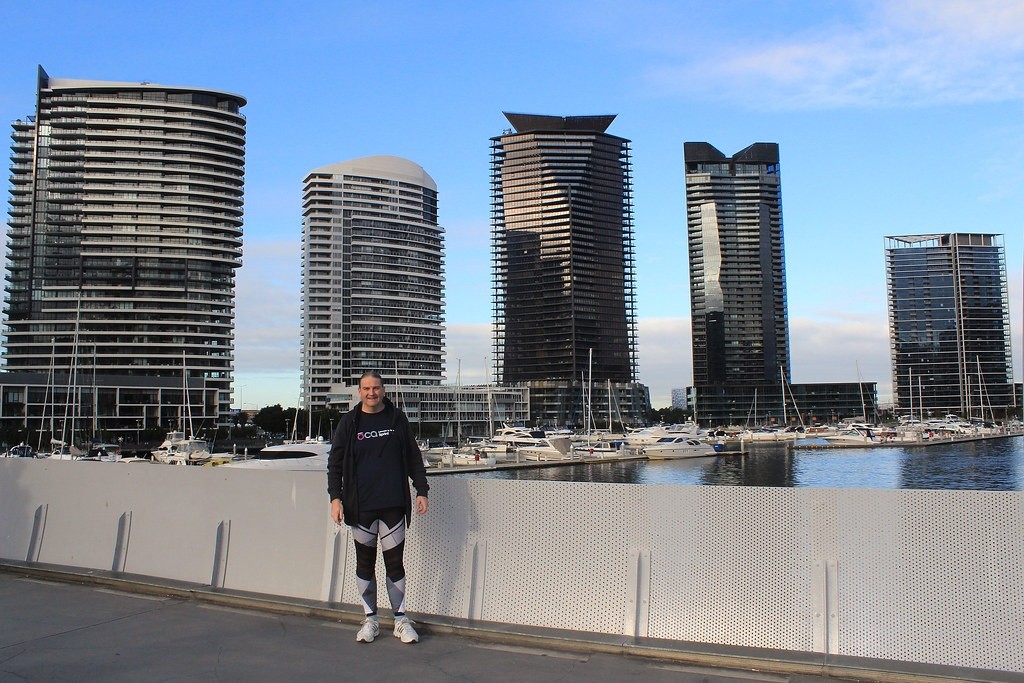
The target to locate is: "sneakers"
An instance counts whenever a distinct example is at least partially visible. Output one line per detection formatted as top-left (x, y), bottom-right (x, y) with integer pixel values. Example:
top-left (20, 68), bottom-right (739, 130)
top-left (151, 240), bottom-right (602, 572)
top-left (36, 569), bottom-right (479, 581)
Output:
top-left (355, 618), bottom-right (379, 643)
top-left (393, 614), bottom-right (419, 643)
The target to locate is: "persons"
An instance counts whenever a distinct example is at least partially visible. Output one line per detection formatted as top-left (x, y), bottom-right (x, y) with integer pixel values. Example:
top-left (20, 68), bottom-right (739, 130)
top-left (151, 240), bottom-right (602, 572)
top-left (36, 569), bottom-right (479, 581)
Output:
top-left (327, 370), bottom-right (430, 643)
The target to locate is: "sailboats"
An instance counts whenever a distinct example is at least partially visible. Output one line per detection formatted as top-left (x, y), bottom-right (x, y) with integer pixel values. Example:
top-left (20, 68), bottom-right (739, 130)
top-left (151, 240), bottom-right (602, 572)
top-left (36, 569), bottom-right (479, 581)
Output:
top-left (387, 344), bottom-right (1006, 470)
top-left (31, 292), bottom-right (121, 462)
top-left (150, 349), bottom-right (235, 466)
